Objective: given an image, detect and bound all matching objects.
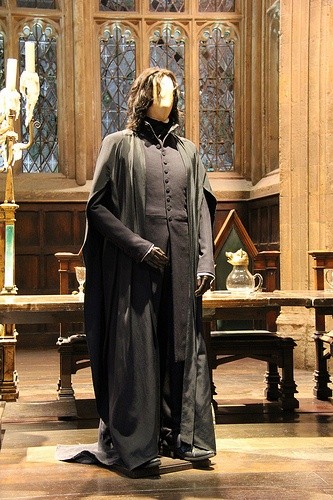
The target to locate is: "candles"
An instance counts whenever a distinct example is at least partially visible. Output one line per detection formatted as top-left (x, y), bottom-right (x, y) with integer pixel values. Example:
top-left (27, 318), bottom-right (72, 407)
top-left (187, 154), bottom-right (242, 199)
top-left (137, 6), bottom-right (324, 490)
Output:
top-left (25, 41), bottom-right (35, 72)
top-left (6, 58), bottom-right (18, 92)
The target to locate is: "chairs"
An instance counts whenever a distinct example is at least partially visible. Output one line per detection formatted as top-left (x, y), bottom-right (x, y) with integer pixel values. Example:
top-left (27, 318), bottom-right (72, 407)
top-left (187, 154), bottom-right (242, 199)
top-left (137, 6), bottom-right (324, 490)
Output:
top-left (54, 252), bottom-right (91, 399)
top-left (205, 209), bottom-right (300, 408)
top-left (309, 251), bottom-right (333, 400)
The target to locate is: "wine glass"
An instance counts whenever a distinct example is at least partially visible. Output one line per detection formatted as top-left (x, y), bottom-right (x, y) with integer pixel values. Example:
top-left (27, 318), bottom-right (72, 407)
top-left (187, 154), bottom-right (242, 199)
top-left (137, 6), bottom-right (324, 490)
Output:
top-left (74, 267), bottom-right (86, 297)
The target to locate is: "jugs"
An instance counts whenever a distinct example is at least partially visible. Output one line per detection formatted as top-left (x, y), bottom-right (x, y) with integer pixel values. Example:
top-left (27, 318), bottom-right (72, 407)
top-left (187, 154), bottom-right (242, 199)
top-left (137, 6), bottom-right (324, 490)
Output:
top-left (225, 247), bottom-right (265, 294)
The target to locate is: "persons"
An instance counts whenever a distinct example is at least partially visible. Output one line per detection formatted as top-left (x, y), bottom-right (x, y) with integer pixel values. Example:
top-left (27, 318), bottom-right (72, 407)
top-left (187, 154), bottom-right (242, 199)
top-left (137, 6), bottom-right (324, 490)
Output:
top-left (78, 67), bottom-right (217, 474)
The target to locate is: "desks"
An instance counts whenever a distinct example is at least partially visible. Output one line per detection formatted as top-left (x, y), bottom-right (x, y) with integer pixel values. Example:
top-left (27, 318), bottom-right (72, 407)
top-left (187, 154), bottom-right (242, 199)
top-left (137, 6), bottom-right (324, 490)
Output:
top-left (0, 289), bottom-right (333, 324)
